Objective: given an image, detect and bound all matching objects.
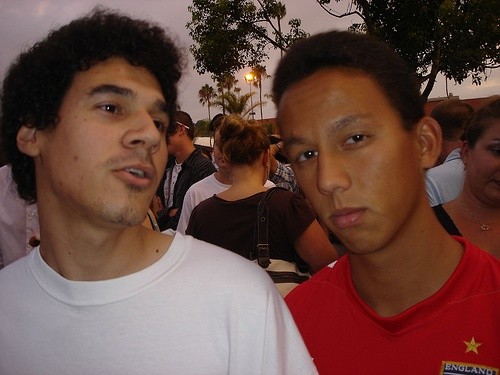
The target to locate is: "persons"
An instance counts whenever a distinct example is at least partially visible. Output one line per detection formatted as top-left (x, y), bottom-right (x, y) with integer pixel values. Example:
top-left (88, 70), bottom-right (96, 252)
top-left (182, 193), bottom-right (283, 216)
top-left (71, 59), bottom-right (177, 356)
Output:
top-left (0, 10), bottom-right (320, 374)
top-left (142, 99), bottom-right (473, 236)
top-left (1, 164), bottom-right (41, 270)
top-left (431, 97), bottom-right (500, 257)
top-left (185, 112), bottom-right (339, 298)
top-left (273, 31), bottom-right (500, 374)
top-left (149, 110), bottom-right (217, 234)
